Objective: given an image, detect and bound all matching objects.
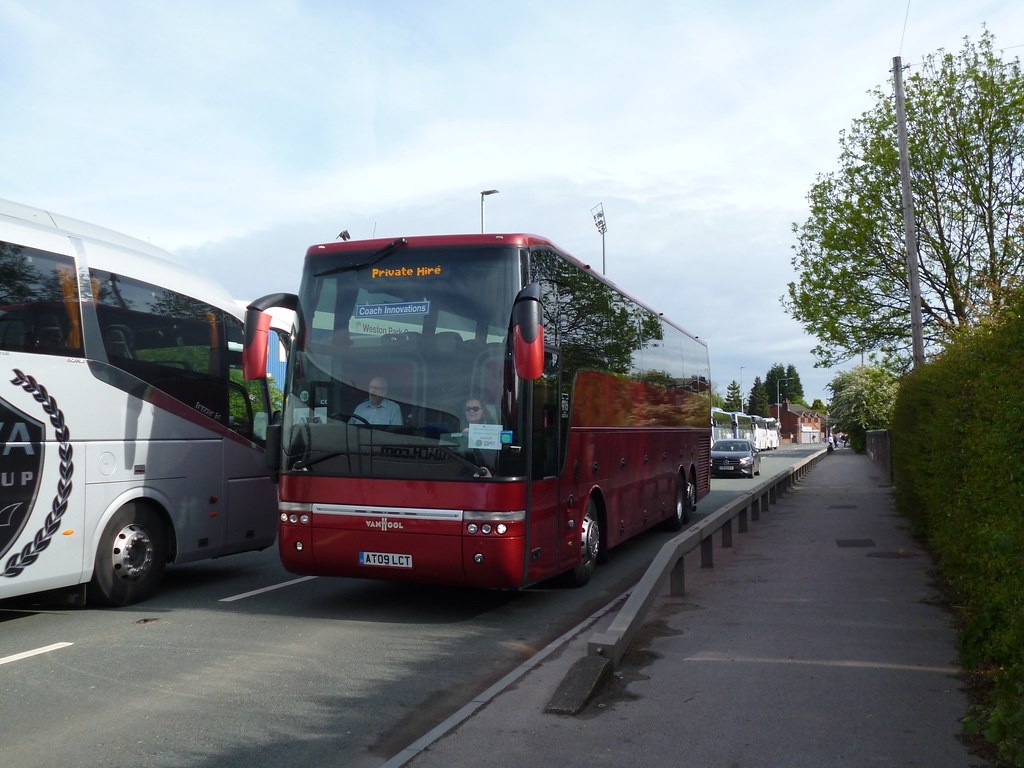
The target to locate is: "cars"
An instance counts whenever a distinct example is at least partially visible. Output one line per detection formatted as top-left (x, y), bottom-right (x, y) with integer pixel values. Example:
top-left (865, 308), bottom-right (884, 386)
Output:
top-left (710, 439), bottom-right (761, 480)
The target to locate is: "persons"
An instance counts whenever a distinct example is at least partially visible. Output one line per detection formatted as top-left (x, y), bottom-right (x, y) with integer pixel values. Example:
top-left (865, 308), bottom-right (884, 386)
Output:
top-left (347, 378), bottom-right (404, 425)
top-left (833, 434), bottom-right (839, 447)
top-left (451, 399), bottom-right (497, 432)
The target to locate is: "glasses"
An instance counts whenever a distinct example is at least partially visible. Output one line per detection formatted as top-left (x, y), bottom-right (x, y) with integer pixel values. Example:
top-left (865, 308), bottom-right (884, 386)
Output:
top-left (464, 407), bottom-right (483, 412)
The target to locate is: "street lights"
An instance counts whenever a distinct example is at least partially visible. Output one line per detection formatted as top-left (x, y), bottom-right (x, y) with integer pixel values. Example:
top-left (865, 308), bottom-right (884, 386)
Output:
top-left (740, 366), bottom-right (746, 413)
top-left (777, 377), bottom-right (795, 426)
top-left (479, 189), bottom-right (499, 233)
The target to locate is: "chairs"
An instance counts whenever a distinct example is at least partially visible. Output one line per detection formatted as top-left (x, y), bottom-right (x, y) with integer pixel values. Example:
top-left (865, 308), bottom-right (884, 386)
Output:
top-left (0, 312), bottom-right (139, 360)
top-left (350, 332), bottom-right (502, 421)
top-left (741, 444), bottom-right (746, 449)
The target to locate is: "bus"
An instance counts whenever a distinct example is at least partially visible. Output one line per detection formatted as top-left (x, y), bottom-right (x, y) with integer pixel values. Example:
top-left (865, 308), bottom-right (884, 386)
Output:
top-left (0, 200), bottom-right (294, 610)
top-left (709, 406), bottom-right (780, 450)
top-left (241, 231), bottom-right (711, 594)
